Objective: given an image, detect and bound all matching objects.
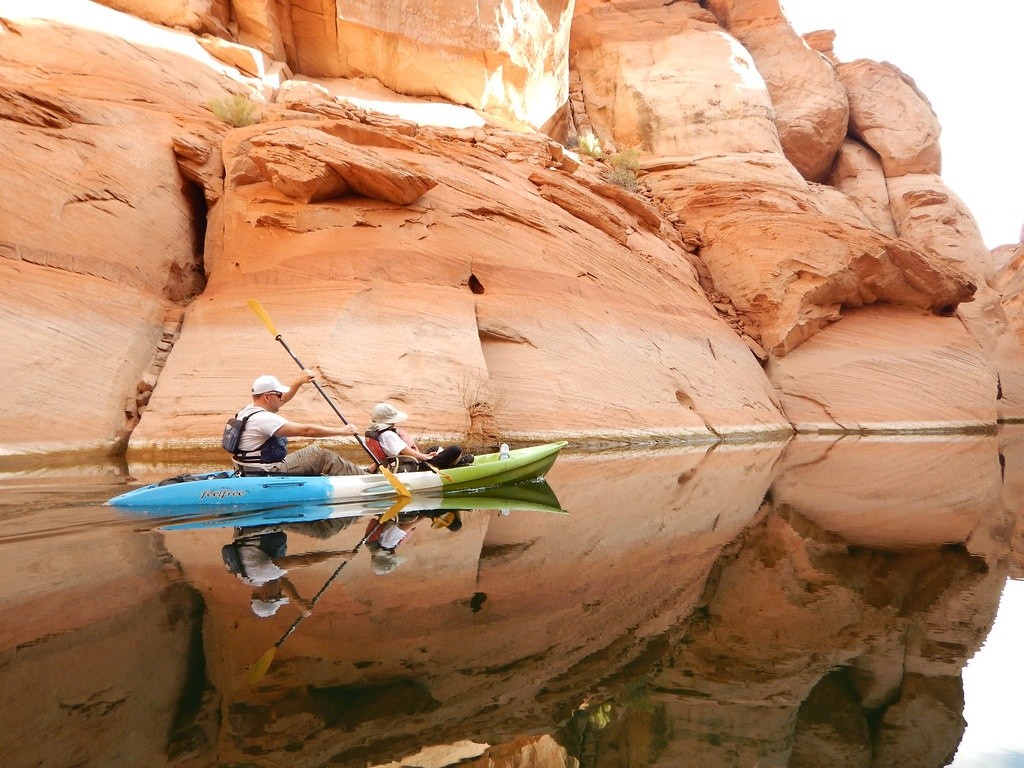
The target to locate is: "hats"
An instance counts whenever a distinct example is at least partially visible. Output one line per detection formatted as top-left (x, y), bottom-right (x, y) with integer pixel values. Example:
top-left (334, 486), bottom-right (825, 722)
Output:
top-left (370, 402), bottom-right (408, 424)
top-left (251, 375), bottom-right (291, 394)
top-left (371, 554), bottom-right (407, 575)
top-left (251, 598), bottom-right (291, 618)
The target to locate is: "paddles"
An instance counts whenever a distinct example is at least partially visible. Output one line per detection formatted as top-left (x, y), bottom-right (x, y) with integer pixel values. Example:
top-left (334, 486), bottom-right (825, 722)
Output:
top-left (236, 500), bottom-right (412, 686)
top-left (245, 294), bottom-right (410, 499)
top-left (424, 464), bottom-right (453, 486)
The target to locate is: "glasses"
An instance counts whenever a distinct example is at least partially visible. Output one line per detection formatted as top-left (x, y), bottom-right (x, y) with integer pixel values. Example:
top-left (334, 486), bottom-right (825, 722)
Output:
top-left (263, 392), bottom-right (282, 398)
top-left (264, 592), bottom-right (282, 604)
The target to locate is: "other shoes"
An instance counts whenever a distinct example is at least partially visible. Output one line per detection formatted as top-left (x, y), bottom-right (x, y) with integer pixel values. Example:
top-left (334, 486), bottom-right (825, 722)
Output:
top-left (363, 459), bottom-right (382, 475)
top-left (458, 453), bottom-right (474, 463)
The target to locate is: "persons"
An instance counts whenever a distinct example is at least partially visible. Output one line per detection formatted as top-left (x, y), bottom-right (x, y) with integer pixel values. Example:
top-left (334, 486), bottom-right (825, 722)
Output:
top-left (232, 512), bottom-right (420, 617)
top-left (365, 509), bottom-right (474, 575)
top-left (365, 403), bottom-right (474, 472)
top-left (233, 369), bottom-right (399, 477)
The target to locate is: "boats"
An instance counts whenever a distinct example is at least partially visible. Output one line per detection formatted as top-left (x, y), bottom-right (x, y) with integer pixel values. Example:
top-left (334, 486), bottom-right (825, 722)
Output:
top-left (111, 479), bottom-right (567, 516)
top-left (104, 441), bottom-right (569, 509)
